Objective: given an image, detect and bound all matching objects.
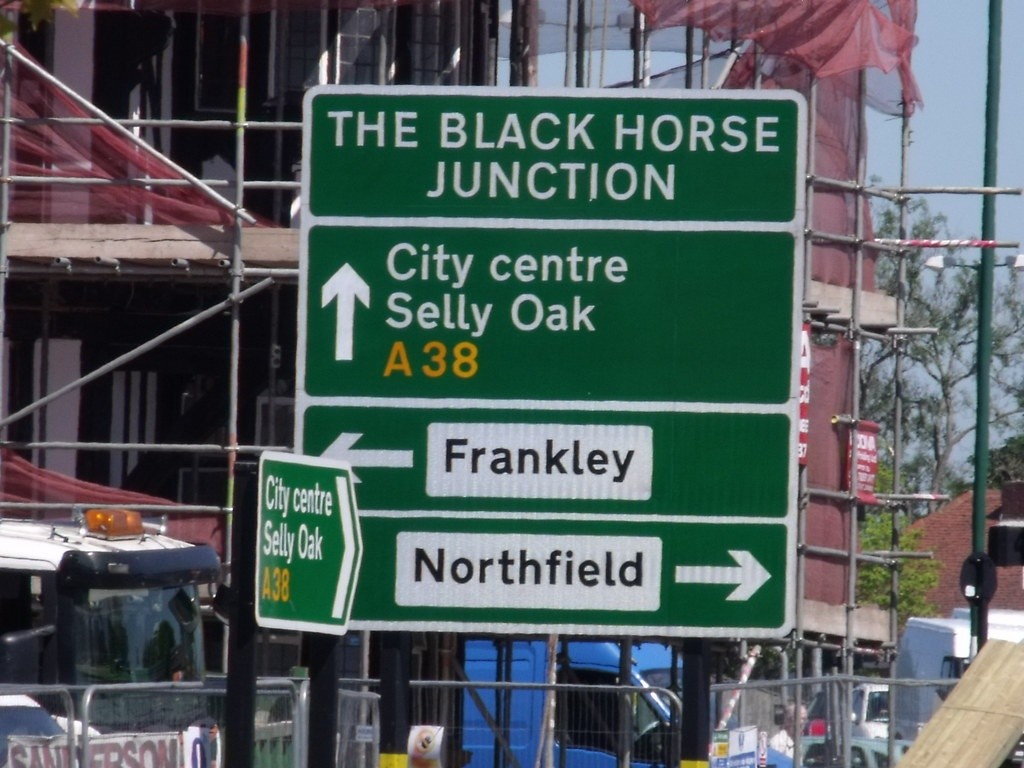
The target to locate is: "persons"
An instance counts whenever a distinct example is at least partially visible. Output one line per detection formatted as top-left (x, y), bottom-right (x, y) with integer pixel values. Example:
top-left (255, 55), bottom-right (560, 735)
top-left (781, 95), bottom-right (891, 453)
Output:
top-left (769, 704), bottom-right (808, 765)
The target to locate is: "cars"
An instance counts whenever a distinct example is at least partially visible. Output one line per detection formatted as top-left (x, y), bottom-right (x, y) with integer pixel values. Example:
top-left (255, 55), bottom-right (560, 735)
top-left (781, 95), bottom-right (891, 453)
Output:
top-left (805, 683), bottom-right (891, 768)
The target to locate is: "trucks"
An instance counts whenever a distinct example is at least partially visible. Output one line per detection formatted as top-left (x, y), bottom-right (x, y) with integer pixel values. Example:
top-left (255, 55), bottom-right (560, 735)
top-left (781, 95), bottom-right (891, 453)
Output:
top-left (454, 637), bottom-right (797, 768)
top-left (0, 510), bottom-right (223, 767)
top-left (888, 609), bottom-right (1024, 762)
